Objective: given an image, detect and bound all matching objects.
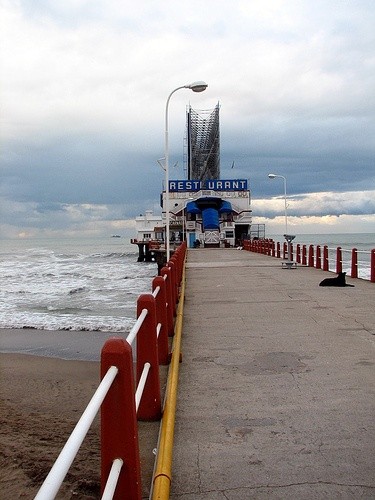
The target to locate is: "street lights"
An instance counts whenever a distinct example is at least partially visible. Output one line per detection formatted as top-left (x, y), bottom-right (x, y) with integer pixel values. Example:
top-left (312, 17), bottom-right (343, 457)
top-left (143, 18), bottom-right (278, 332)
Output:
top-left (164, 82), bottom-right (208, 264)
top-left (182, 197), bottom-right (193, 242)
top-left (267, 173), bottom-right (287, 234)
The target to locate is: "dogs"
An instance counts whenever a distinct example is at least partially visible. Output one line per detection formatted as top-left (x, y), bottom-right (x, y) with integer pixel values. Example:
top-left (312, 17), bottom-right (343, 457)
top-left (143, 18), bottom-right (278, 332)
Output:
top-left (319, 272), bottom-right (355, 287)
